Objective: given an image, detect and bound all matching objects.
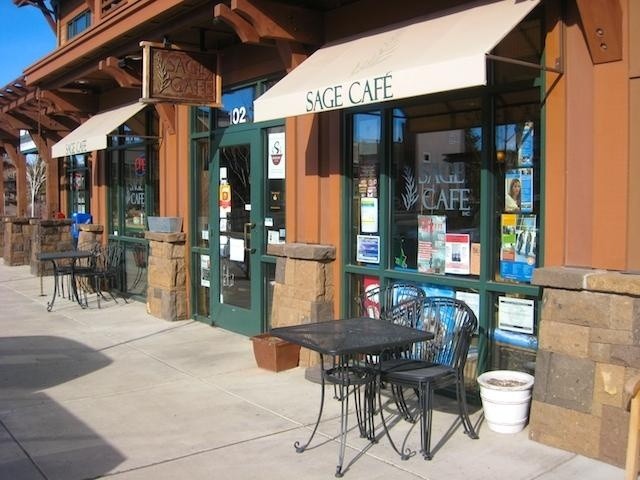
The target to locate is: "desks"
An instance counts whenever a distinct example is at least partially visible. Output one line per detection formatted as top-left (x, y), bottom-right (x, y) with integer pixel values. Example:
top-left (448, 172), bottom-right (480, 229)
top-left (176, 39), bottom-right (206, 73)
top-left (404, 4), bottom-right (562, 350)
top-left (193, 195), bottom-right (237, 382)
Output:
top-left (270, 317), bottom-right (436, 477)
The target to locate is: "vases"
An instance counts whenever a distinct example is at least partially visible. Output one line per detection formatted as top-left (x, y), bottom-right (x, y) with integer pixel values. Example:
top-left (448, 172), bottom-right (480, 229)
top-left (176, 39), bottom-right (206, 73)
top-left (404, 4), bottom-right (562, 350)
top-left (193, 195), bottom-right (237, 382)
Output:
top-left (476, 366), bottom-right (535, 437)
top-left (247, 330), bottom-right (303, 376)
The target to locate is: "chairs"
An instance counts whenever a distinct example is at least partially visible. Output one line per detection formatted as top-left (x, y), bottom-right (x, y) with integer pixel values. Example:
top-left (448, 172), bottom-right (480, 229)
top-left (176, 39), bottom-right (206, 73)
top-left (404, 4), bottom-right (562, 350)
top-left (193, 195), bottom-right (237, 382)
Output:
top-left (37, 239), bottom-right (125, 310)
top-left (327, 281), bottom-right (482, 463)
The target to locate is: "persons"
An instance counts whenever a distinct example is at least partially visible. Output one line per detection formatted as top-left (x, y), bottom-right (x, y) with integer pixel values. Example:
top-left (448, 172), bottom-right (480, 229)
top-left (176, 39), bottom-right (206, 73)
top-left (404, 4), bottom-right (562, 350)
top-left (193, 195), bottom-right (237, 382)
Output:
top-left (505, 179), bottom-right (521, 213)
top-left (517, 226), bottom-right (531, 257)
